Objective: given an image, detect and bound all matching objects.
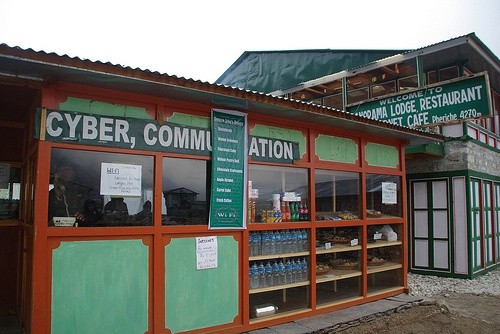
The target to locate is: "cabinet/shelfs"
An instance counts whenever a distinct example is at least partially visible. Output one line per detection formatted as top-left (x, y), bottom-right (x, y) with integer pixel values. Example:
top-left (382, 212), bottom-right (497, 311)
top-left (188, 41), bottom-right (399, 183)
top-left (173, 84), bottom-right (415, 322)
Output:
top-left (249, 212), bottom-right (403, 324)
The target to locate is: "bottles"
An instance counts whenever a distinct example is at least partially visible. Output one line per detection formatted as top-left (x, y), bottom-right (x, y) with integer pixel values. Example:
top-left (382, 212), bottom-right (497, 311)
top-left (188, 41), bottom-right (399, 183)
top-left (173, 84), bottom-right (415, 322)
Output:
top-left (281, 201), bottom-right (308, 222)
top-left (249, 229), bottom-right (307, 256)
top-left (274, 210), bottom-right (282, 223)
top-left (249, 256), bottom-right (308, 289)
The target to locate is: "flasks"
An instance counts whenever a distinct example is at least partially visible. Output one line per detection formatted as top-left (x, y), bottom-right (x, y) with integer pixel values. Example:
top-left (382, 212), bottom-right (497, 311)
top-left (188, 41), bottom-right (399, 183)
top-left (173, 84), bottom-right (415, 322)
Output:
top-left (252, 303), bottom-right (279, 318)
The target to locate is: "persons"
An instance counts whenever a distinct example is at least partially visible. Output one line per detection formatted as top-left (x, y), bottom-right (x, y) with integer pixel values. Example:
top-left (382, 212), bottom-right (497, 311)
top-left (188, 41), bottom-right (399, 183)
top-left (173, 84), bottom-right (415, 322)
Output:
top-left (136, 199), bottom-right (153, 226)
top-left (48, 163), bottom-right (95, 228)
top-left (104, 196), bottom-right (130, 226)
